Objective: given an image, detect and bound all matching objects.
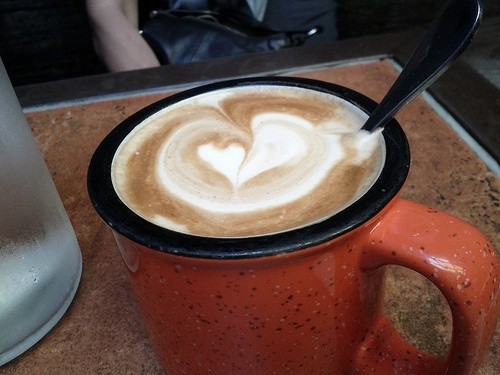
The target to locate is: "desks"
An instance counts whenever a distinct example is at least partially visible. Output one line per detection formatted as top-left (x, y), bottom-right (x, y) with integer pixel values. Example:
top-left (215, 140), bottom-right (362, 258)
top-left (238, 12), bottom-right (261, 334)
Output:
top-left (0, 14), bottom-right (500, 374)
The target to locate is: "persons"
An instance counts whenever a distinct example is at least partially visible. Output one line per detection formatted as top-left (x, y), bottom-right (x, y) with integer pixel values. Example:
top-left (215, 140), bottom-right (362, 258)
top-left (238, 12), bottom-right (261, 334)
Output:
top-left (86, 0), bottom-right (338, 72)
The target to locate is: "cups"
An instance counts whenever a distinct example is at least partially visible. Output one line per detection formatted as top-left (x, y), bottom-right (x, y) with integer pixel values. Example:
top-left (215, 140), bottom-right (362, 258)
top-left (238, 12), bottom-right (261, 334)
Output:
top-left (0, 58), bottom-right (83, 365)
top-left (87, 76), bottom-right (500, 375)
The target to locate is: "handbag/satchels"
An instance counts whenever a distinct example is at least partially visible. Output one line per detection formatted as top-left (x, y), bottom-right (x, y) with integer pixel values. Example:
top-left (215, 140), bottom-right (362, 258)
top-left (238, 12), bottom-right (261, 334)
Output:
top-left (139, 7), bottom-right (324, 67)
